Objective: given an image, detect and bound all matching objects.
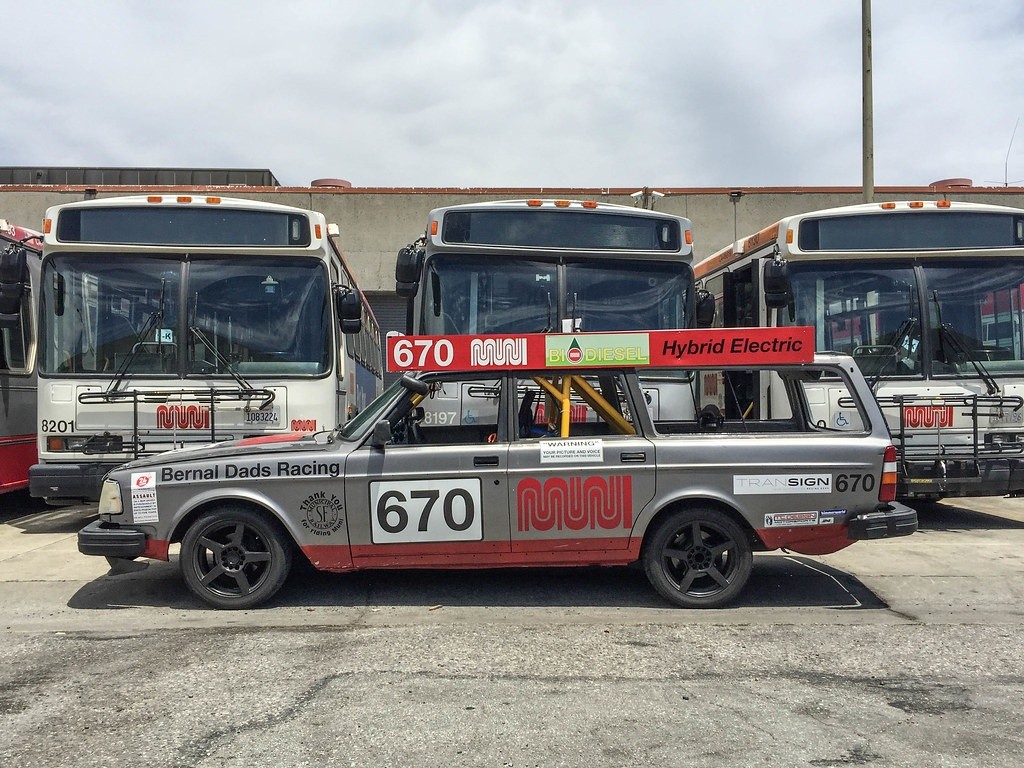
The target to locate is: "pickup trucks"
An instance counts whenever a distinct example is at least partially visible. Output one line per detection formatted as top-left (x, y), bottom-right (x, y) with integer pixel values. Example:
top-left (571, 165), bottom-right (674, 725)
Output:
top-left (73, 323), bottom-right (920, 608)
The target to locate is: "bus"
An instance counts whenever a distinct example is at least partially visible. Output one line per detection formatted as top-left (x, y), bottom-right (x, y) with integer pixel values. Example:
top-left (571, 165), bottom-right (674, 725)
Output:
top-left (28, 188), bottom-right (383, 504)
top-left (394, 198), bottom-right (705, 422)
top-left (687, 200), bottom-right (1023, 500)
top-left (2, 218), bottom-right (47, 494)
